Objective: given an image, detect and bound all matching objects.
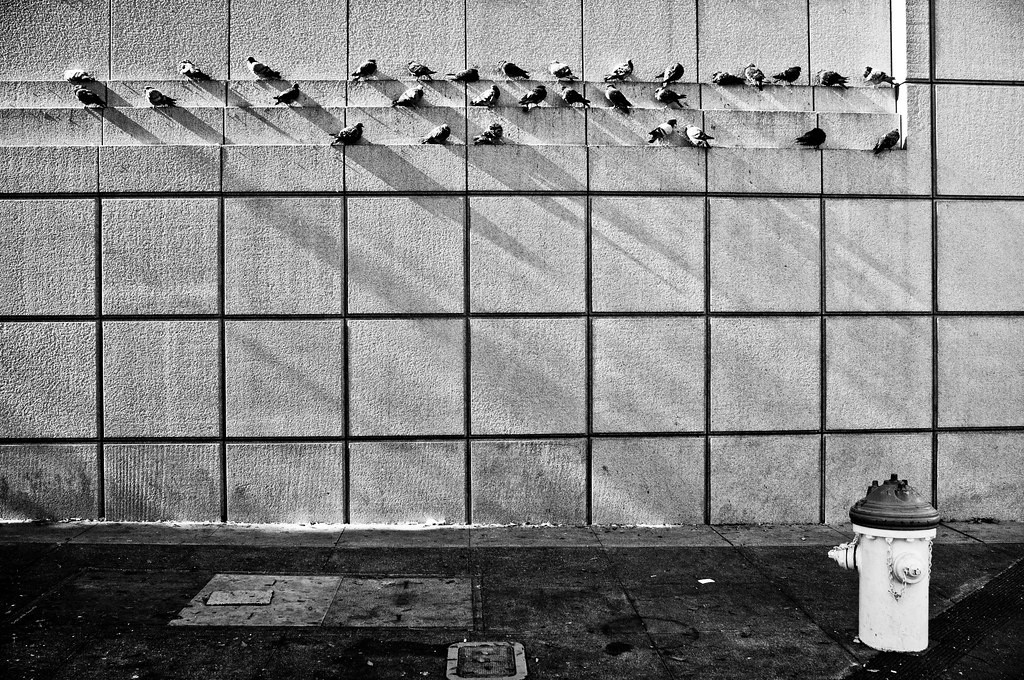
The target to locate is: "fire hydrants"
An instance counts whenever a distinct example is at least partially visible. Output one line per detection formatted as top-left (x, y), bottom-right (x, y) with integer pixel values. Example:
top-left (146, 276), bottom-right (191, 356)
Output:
top-left (828, 472), bottom-right (942, 660)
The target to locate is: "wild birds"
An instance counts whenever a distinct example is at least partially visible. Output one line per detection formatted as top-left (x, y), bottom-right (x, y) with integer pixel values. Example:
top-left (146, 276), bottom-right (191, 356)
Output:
top-left (446, 66), bottom-right (503, 146)
top-left (329, 123), bottom-right (364, 145)
top-left (180, 60), bottom-right (210, 81)
top-left (646, 64), bottom-right (714, 150)
top-left (604, 58), bottom-right (634, 114)
top-left (64, 69), bottom-right (108, 109)
top-left (713, 71), bottom-right (744, 87)
top-left (865, 73), bottom-right (900, 155)
top-left (390, 60), bottom-right (450, 144)
top-left (794, 128), bottom-right (827, 147)
top-left (746, 63), bottom-right (850, 90)
top-left (550, 60), bottom-right (591, 108)
top-left (351, 58), bottom-right (377, 83)
top-left (497, 60), bottom-right (547, 111)
top-left (245, 56), bottom-right (300, 104)
top-left (143, 86), bottom-right (177, 110)
